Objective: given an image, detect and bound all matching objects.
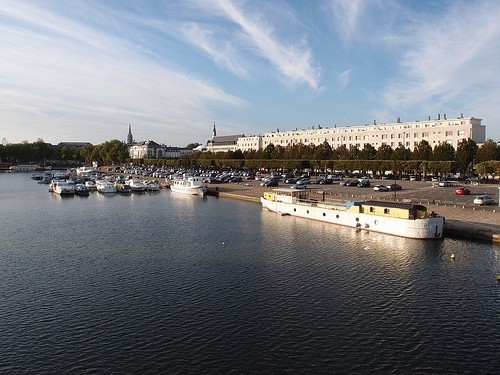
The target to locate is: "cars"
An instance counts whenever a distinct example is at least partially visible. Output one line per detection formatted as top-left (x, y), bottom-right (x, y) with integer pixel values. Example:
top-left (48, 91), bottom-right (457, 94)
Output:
top-left (392, 174), bottom-right (439, 182)
top-left (373, 184), bottom-right (389, 192)
top-left (438, 180), bottom-right (452, 187)
top-left (114, 163), bottom-right (373, 188)
top-left (466, 176), bottom-right (479, 183)
top-left (473, 194), bottom-right (495, 206)
top-left (386, 183), bottom-right (399, 191)
top-left (382, 175), bottom-right (388, 180)
top-left (373, 174), bottom-right (380, 179)
top-left (455, 187), bottom-right (471, 195)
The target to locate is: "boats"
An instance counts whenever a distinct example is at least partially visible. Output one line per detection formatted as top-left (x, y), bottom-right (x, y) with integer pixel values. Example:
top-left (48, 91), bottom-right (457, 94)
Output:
top-left (9, 164), bottom-right (41, 173)
top-left (169, 170), bottom-right (220, 197)
top-left (261, 186), bottom-right (446, 241)
top-left (30, 170), bottom-right (161, 197)
